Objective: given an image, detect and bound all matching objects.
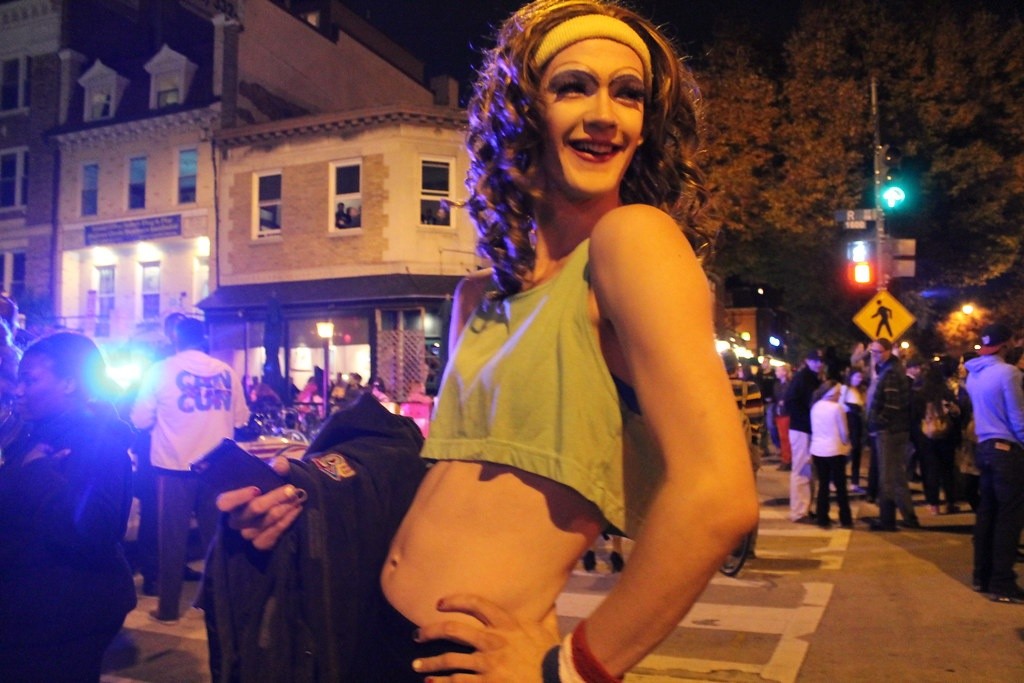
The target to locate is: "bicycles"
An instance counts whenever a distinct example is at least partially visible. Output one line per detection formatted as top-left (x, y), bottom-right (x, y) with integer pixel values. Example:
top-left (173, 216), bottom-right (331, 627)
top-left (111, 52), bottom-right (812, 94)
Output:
top-left (714, 528), bottom-right (754, 575)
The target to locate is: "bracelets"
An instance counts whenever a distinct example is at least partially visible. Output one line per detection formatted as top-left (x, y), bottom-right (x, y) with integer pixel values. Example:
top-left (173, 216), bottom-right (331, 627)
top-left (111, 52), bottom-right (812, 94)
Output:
top-left (541, 619), bottom-right (625, 683)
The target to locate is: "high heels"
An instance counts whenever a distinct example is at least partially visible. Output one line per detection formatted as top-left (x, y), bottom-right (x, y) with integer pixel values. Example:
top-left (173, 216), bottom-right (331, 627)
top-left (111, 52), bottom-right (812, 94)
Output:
top-left (584, 550), bottom-right (596, 573)
top-left (610, 551), bottom-right (622, 574)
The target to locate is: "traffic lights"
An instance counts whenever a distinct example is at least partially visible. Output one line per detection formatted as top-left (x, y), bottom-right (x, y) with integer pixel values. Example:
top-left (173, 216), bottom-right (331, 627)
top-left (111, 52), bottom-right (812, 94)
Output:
top-left (881, 182), bottom-right (908, 211)
top-left (844, 239), bottom-right (875, 287)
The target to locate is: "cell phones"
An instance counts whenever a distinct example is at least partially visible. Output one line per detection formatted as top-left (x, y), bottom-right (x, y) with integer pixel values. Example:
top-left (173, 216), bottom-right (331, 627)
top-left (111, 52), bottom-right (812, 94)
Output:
top-left (190, 437), bottom-right (286, 495)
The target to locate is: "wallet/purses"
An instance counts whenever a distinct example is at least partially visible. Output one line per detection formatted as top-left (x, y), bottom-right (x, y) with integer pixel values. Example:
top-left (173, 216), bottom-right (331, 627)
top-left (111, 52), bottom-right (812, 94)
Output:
top-left (188, 438), bottom-right (308, 511)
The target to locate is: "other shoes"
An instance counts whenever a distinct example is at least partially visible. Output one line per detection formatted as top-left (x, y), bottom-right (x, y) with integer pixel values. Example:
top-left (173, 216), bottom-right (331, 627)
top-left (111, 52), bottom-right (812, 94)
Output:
top-left (928, 505), bottom-right (940, 515)
top-left (990, 586), bottom-right (1024, 604)
top-left (946, 505), bottom-right (960, 513)
top-left (1015, 544), bottom-right (1024, 562)
top-left (143, 582), bottom-right (159, 595)
top-left (183, 566), bottom-right (203, 581)
top-left (148, 610), bottom-right (178, 624)
top-left (973, 571), bottom-right (1018, 590)
top-left (187, 604), bottom-right (206, 618)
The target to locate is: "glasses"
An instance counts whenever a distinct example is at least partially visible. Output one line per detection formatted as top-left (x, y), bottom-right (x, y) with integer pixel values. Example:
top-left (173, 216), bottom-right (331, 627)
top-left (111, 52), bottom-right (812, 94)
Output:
top-left (868, 349), bottom-right (886, 355)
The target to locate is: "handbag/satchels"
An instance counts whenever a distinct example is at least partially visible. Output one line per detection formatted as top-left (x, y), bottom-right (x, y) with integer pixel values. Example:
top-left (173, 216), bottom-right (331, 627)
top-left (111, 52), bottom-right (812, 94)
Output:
top-left (921, 400), bottom-right (961, 439)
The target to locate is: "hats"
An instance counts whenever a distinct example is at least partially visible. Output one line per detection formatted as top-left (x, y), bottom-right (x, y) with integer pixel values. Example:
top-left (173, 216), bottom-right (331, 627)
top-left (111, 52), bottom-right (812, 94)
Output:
top-left (977, 323), bottom-right (1014, 355)
top-left (806, 350), bottom-right (827, 360)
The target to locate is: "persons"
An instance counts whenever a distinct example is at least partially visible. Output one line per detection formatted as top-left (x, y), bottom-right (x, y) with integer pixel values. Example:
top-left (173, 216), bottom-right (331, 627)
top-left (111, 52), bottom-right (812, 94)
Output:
top-left (0, 296), bottom-right (437, 683)
top-left (721, 327), bottom-right (1024, 603)
top-left (215, 0), bottom-right (759, 683)
